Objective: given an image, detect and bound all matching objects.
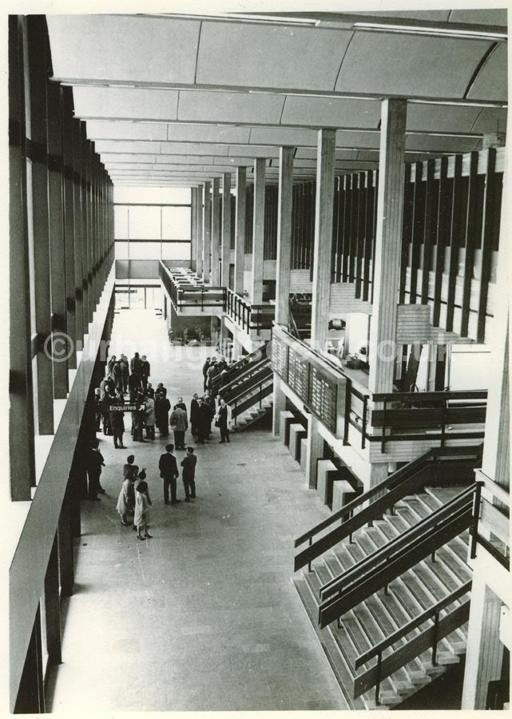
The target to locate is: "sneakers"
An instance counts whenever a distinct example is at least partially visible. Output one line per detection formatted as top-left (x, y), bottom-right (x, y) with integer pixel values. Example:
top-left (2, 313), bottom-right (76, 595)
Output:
top-left (120, 521), bottom-right (152, 541)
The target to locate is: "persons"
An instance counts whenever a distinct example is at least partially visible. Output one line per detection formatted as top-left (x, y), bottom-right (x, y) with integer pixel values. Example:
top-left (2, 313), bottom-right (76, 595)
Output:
top-left (88, 353), bottom-right (232, 541)
